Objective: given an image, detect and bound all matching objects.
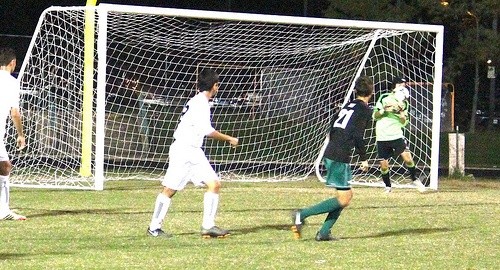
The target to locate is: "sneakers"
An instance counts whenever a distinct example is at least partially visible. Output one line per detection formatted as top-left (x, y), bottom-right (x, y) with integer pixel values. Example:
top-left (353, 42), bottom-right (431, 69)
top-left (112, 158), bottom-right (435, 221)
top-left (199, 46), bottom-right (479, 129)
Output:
top-left (315, 231), bottom-right (340, 241)
top-left (146, 227), bottom-right (174, 238)
top-left (0, 210), bottom-right (27, 220)
top-left (200, 226), bottom-right (231, 239)
top-left (384, 184), bottom-right (392, 194)
top-left (413, 177), bottom-right (426, 193)
top-left (291, 208), bottom-right (305, 240)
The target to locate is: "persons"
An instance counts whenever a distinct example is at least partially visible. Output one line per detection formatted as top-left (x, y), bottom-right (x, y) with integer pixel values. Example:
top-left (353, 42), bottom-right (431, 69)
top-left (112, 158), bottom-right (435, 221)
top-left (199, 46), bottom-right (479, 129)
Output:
top-left (0, 48), bottom-right (28, 223)
top-left (370, 76), bottom-right (428, 194)
top-left (148, 69), bottom-right (240, 241)
top-left (293, 78), bottom-right (374, 239)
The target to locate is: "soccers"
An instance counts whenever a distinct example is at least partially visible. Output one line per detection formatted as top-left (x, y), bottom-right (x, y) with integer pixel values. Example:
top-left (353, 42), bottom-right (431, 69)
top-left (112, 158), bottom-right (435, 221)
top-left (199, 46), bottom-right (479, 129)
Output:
top-left (393, 86), bottom-right (410, 101)
